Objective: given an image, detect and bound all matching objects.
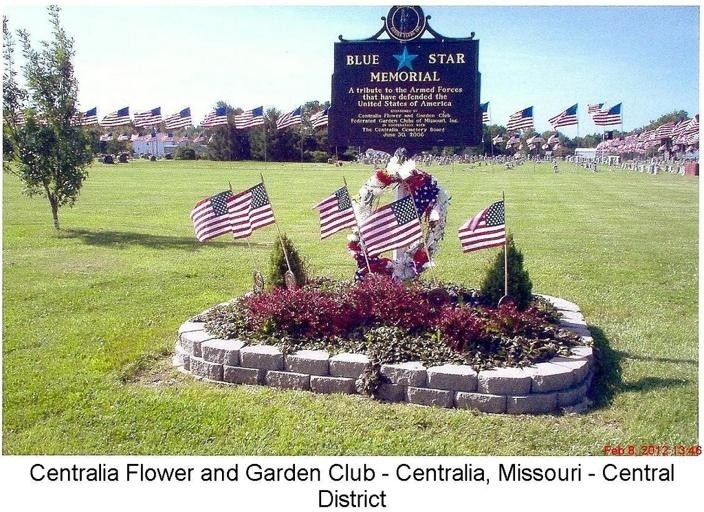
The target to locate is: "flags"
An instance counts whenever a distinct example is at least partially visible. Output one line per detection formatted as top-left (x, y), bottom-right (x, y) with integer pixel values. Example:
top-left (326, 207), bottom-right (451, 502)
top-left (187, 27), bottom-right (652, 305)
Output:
top-left (97, 131), bottom-right (217, 145)
top-left (356, 194), bottom-right (422, 257)
top-left (480, 102), bottom-right (489, 123)
top-left (586, 103), bottom-right (600, 114)
top-left (316, 185), bottom-right (358, 241)
top-left (488, 114), bottom-right (699, 152)
top-left (505, 105), bottom-right (532, 132)
top-left (592, 102), bottom-right (622, 127)
top-left (3, 101), bottom-right (329, 131)
top-left (457, 200), bottom-right (505, 254)
top-left (189, 187), bottom-right (236, 242)
top-left (226, 180), bottom-right (275, 241)
top-left (547, 103), bottom-right (577, 132)
